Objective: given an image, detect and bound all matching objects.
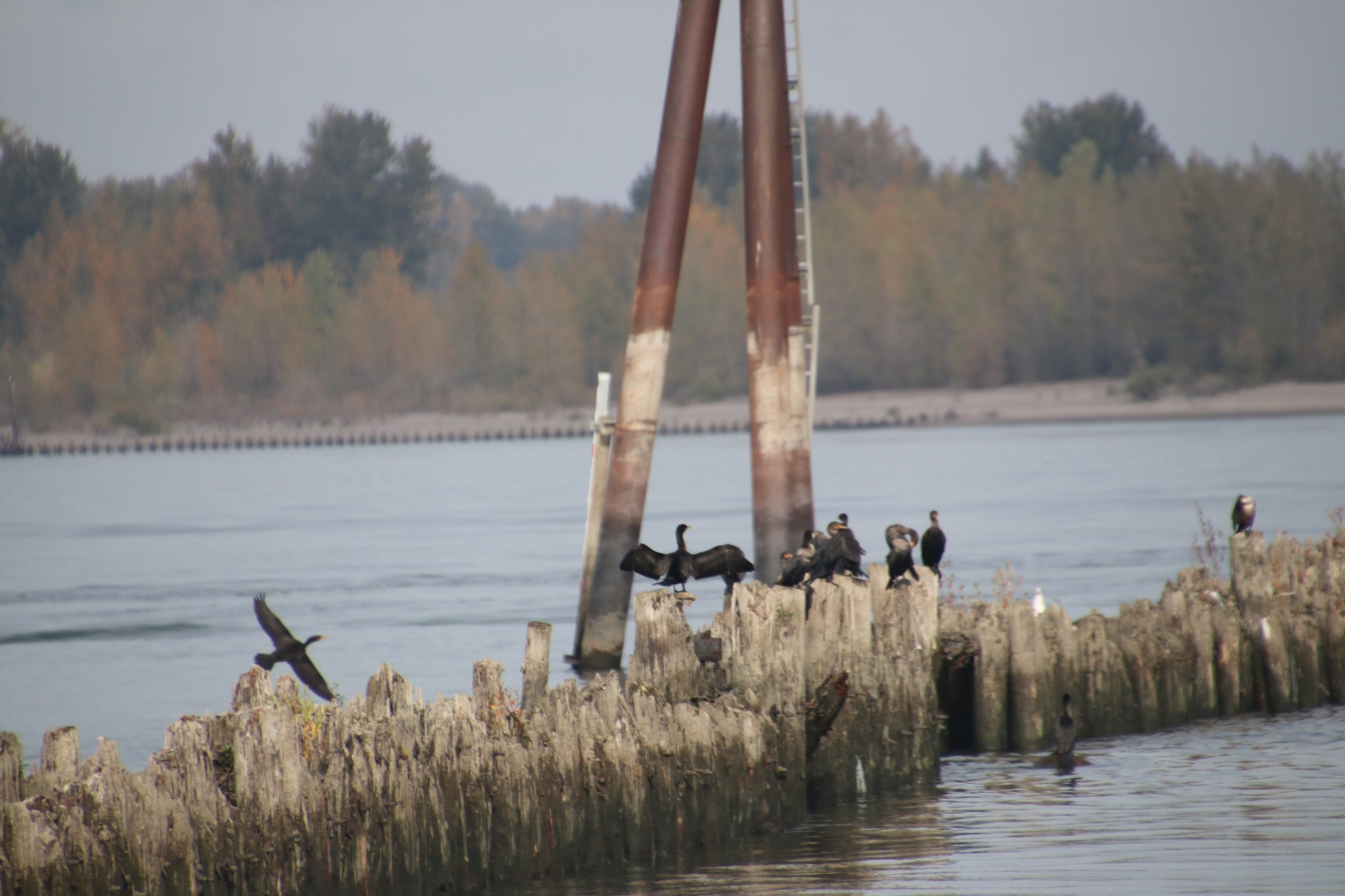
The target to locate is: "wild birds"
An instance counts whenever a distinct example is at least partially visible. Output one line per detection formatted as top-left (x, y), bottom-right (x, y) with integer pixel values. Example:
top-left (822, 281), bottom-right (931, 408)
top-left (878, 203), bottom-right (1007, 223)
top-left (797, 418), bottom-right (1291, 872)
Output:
top-left (885, 525), bottom-right (918, 590)
top-left (1233, 493), bottom-right (1256, 533)
top-left (921, 512), bottom-right (947, 579)
top-left (1054, 692), bottom-right (1076, 757)
top-left (619, 524), bottom-right (755, 596)
top-left (776, 512), bottom-right (869, 587)
top-left (252, 591), bottom-right (336, 702)
